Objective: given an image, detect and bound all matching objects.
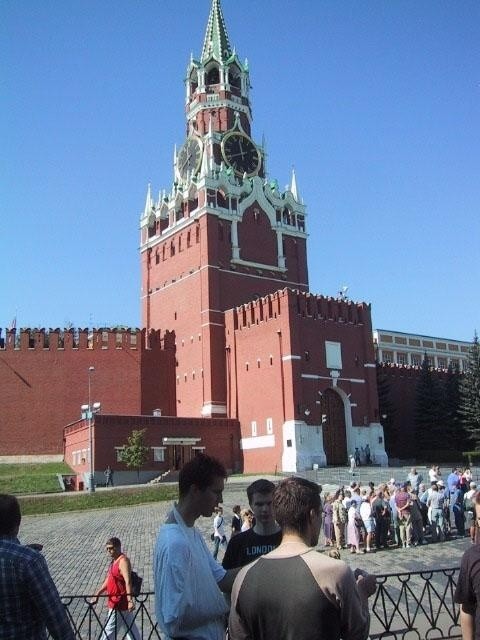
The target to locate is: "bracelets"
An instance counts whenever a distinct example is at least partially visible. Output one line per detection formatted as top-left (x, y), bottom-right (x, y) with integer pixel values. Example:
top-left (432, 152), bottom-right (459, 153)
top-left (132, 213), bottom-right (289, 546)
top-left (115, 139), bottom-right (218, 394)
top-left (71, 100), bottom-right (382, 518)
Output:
top-left (128, 600), bottom-right (133, 603)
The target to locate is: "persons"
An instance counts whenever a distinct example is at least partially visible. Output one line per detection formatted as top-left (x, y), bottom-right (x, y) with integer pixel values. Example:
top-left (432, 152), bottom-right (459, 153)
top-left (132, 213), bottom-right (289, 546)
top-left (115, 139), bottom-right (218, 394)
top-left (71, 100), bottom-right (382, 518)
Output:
top-left (104, 466), bottom-right (115, 488)
top-left (0, 494), bottom-right (74, 640)
top-left (229, 474), bottom-right (378, 640)
top-left (454, 490), bottom-right (480, 639)
top-left (213, 443), bottom-right (480, 569)
top-left (90, 537), bottom-right (141, 640)
top-left (152, 453), bottom-right (247, 640)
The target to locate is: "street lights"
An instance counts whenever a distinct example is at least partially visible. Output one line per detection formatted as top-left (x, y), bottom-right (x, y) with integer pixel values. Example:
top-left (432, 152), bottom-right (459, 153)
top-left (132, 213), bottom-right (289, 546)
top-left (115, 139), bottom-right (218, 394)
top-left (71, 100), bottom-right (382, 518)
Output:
top-left (87, 365), bottom-right (96, 493)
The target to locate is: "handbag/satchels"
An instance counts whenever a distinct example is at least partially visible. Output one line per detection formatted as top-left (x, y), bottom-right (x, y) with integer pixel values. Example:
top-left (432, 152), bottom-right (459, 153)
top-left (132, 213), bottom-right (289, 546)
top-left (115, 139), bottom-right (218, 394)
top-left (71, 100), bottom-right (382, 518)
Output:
top-left (211, 533), bottom-right (215, 541)
top-left (128, 572), bottom-right (144, 597)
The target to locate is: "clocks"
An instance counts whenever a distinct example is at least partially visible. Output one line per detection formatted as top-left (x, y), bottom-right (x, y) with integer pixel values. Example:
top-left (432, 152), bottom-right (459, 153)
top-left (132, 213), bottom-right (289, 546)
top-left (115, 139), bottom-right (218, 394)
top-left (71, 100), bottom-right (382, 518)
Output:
top-left (220, 130), bottom-right (262, 177)
top-left (176, 134), bottom-right (203, 182)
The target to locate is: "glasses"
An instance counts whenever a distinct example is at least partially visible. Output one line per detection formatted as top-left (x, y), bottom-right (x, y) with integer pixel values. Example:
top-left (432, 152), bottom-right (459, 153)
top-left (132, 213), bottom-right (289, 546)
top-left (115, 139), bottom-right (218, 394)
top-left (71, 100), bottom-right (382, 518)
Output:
top-left (107, 547), bottom-right (115, 550)
